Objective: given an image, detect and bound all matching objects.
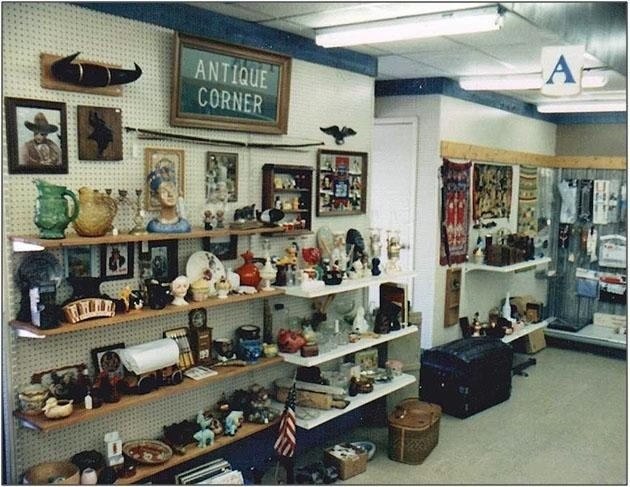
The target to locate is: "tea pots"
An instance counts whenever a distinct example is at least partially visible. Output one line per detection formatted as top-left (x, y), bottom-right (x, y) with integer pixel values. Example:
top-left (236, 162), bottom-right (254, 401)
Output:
top-left (71, 187), bottom-right (119, 238)
top-left (32, 179), bottom-right (80, 239)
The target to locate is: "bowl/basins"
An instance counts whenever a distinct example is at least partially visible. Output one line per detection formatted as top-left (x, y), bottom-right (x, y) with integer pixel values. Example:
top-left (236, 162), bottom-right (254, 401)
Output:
top-left (238, 324), bottom-right (260, 340)
top-left (22, 461), bottom-right (80, 486)
top-left (351, 440), bottom-right (376, 461)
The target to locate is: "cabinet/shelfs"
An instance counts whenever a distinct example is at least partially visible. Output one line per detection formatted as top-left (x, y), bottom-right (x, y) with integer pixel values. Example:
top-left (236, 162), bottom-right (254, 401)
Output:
top-left (458, 255), bottom-right (552, 345)
top-left (5, 199), bottom-right (290, 485)
top-left (260, 163), bottom-right (315, 237)
top-left (260, 270), bottom-right (419, 431)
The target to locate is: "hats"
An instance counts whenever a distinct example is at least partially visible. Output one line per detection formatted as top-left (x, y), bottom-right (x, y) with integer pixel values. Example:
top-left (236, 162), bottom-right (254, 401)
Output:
top-left (24, 113), bottom-right (58, 133)
top-left (111, 248), bottom-right (120, 253)
top-left (147, 168), bottom-right (175, 190)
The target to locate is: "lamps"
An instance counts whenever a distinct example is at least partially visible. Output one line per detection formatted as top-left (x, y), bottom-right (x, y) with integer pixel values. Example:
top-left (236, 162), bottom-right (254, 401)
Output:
top-left (312, 2), bottom-right (504, 50)
top-left (458, 70), bottom-right (608, 91)
top-left (536, 99), bottom-right (627, 114)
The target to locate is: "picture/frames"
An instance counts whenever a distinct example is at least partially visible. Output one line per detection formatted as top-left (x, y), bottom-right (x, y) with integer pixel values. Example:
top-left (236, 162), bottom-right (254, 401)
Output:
top-left (170, 32), bottom-right (292, 136)
top-left (205, 151), bottom-right (239, 203)
top-left (5, 93), bottom-right (68, 177)
top-left (142, 146), bottom-right (186, 213)
top-left (141, 241), bottom-right (179, 284)
top-left (76, 104), bottom-right (125, 163)
top-left (100, 243), bottom-right (135, 282)
top-left (63, 245), bottom-right (96, 279)
top-left (90, 342), bottom-right (129, 380)
top-left (316, 147), bottom-right (369, 217)
top-left (203, 235), bottom-right (238, 260)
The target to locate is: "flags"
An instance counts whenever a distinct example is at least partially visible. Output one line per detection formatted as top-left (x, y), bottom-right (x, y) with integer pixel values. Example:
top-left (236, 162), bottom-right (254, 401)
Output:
top-left (274, 382), bottom-right (298, 458)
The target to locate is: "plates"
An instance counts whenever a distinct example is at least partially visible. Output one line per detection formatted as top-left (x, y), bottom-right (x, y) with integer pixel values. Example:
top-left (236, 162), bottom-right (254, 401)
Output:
top-left (19, 253), bottom-right (64, 293)
top-left (186, 250), bottom-right (227, 297)
top-left (123, 439), bottom-right (173, 464)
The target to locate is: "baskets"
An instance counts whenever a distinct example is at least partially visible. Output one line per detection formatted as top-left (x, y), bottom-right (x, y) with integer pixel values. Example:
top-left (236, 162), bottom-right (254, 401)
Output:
top-left (387, 397), bottom-right (442, 467)
top-left (323, 442), bottom-right (368, 481)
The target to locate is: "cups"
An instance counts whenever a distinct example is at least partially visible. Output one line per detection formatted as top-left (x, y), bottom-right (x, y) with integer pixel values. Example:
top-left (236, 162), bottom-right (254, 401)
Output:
top-left (81, 467), bottom-right (98, 485)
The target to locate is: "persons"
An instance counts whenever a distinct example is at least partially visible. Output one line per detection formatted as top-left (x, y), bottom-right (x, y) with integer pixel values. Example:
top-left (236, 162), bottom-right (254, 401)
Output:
top-left (170, 275), bottom-right (189, 305)
top-left (145, 168), bottom-right (191, 233)
top-left (153, 248), bottom-right (166, 277)
top-left (109, 248), bottom-right (125, 271)
top-left (21, 112), bottom-right (60, 166)
